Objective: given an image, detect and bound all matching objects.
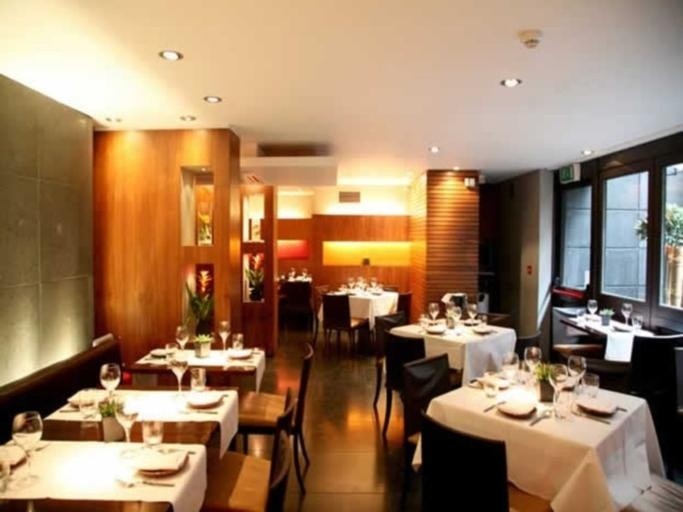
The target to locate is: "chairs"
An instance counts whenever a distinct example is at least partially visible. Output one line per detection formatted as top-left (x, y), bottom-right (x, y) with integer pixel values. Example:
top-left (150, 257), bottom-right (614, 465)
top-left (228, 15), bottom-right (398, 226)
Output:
top-left (201, 399), bottom-right (296, 511)
top-left (323, 294), bottom-right (369, 360)
top-left (396, 293), bottom-right (412, 315)
top-left (373, 316), bottom-right (509, 511)
top-left (600, 335), bottom-right (683, 402)
top-left (277, 282), bottom-right (313, 343)
top-left (550, 306), bottom-right (602, 365)
top-left (619, 476), bottom-right (683, 512)
top-left (237, 344), bottom-right (315, 493)
top-left (515, 332), bottom-right (541, 362)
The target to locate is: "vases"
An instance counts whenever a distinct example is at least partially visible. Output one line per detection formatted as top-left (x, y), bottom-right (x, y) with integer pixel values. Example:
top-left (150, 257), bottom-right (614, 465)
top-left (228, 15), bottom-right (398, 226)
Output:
top-left (193, 321), bottom-right (211, 335)
top-left (248, 289), bottom-right (263, 300)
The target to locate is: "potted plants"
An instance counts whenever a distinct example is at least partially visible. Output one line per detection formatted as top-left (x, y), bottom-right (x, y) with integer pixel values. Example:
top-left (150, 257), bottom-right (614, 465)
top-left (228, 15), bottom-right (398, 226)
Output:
top-left (600, 309), bottom-right (615, 326)
top-left (534, 363), bottom-right (562, 402)
top-left (193, 334), bottom-right (214, 357)
top-left (98, 399), bottom-right (124, 441)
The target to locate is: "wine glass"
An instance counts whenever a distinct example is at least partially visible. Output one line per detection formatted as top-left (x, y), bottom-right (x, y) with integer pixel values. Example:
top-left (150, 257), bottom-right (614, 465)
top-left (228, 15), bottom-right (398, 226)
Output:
top-left (429, 301), bottom-right (479, 331)
top-left (11, 320), bottom-right (231, 489)
top-left (576, 299), bottom-right (633, 334)
top-left (347, 276), bottom-right (377, 293)
top-left (483, 346), bottom-right (599, 421)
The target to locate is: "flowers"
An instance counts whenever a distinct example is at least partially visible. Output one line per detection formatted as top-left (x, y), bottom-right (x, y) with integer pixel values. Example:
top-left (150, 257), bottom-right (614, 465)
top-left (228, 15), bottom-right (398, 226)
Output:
top-left (244, 255), bottom-right (267, 287)
top-left (182, 271), bottom-right (216, 318)
